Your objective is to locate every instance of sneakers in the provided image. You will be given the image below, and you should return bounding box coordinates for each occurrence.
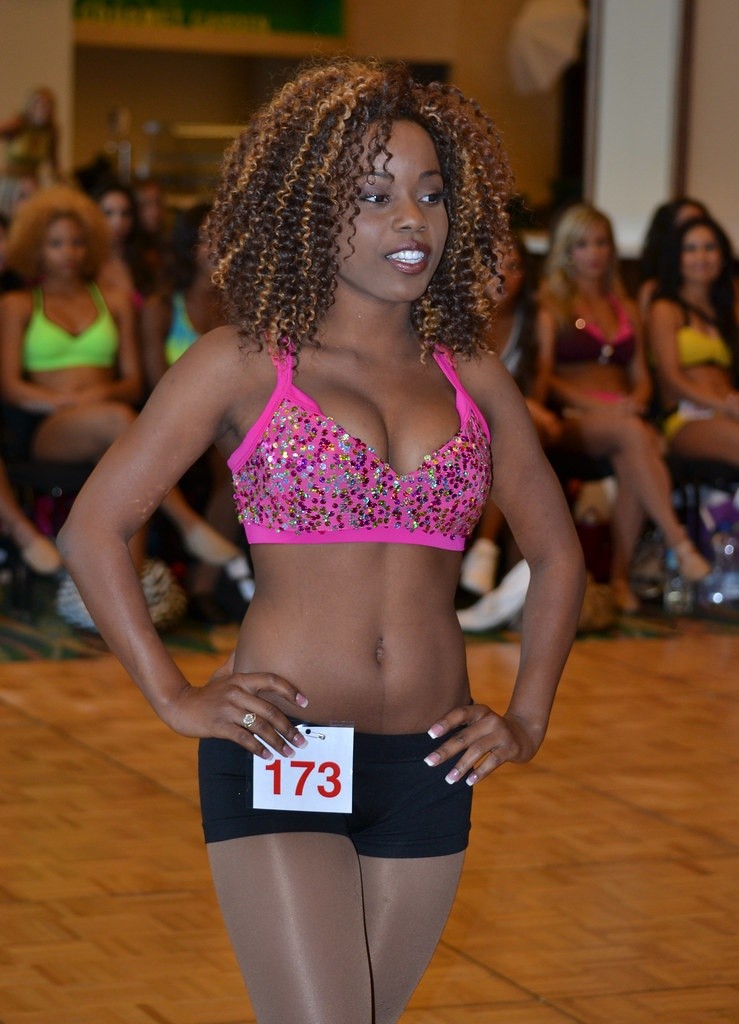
[460,539,500,594]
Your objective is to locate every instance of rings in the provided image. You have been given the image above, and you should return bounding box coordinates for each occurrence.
[243,714,254,726]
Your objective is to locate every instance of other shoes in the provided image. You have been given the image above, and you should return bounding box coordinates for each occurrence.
[183,524,240,566]
[19,533,63,576]
[606,576,640,614]
[666,532,708,581]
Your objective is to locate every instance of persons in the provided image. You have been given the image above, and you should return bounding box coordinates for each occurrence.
[0,86,248,631]
[53,65,587,1023]
[460,197,739,615]
